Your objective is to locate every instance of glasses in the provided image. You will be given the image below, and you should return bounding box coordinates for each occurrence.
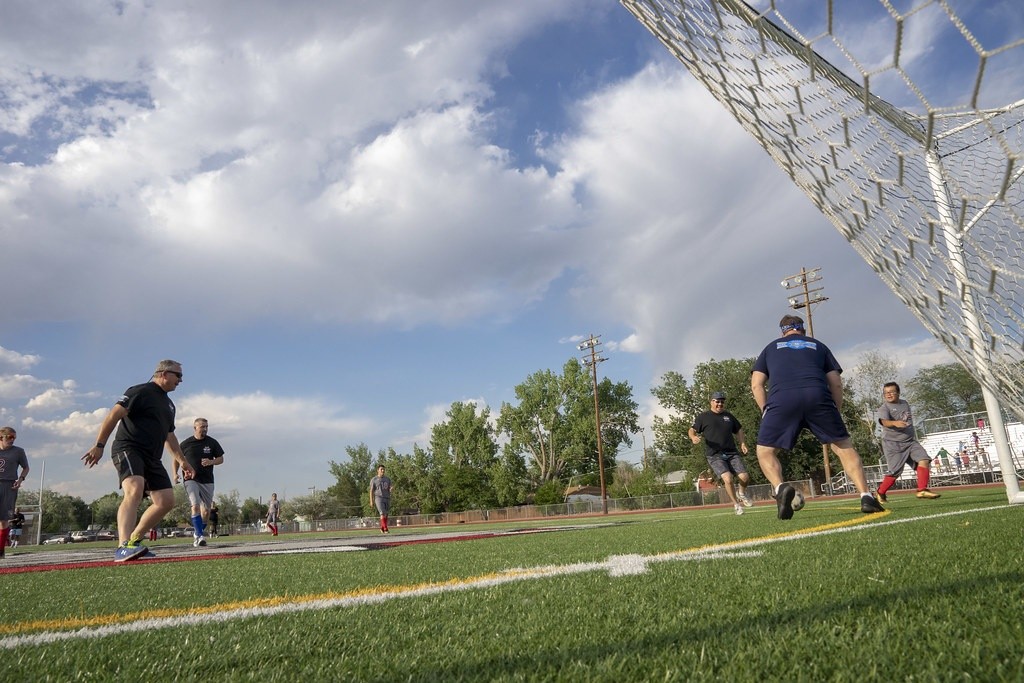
[159,369,183,378]
[884,390,898,396]
[1,434,16,440]
[712,400,724,404]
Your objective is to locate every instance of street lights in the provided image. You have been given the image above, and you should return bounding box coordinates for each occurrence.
[576,333,610,515]
[780,265,833,488]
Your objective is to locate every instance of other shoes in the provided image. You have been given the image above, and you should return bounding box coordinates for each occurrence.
[272,528,275,533]
[380,528,385,533]
[271,533,278,536]
[11,543,14,548]
[384,529,389,533]
[0,550,5,559]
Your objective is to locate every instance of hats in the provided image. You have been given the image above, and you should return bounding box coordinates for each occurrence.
[711,391,726,400]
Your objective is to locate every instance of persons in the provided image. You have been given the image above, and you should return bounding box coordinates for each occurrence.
[688,391,753,515]
[209,501,219,538]
[80,359,196,562]
[173,418,225,547]
[8,507,25,548]
[750,315,884,521]
[265,493,280,536]
[978,418,983,429]
[934,431,984,472]
[369,465,393,534]
[149,527,157,541]
[0,427,29,559]
[875,382,941,503]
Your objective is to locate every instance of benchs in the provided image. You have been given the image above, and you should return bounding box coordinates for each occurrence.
[882,422,1024,480]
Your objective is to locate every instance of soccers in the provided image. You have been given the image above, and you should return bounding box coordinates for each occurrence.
[791,490,804,511]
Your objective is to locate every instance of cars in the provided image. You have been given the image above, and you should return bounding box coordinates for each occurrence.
[42,535,75,544]
[67,530,119,542]
[144,526,195,539]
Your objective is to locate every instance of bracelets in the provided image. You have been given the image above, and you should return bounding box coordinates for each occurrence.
[20,476,25,481]
[96,443,104,448]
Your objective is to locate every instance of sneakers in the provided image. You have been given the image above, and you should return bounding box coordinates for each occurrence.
[875,489,887,504]
[198,535,207,546]
[736,490,753,506]
[734,505,744,515]
[861,493,885,512]
[915,489,941,500]
[128,538,156,559]
[114,540,148,562]
[193,532,199,547]
[770,483,796,520]
